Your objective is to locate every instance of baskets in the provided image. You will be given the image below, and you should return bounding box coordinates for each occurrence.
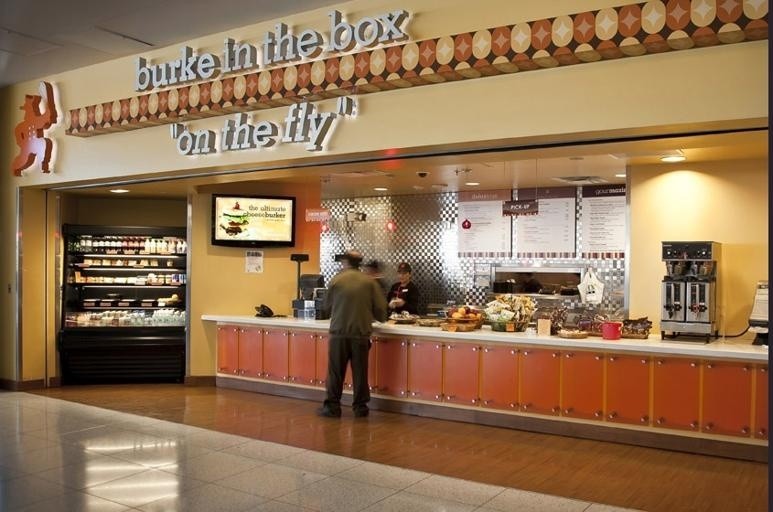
[439,311,487,332]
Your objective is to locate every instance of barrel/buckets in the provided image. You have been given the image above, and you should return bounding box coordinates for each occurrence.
[602,321,624,340]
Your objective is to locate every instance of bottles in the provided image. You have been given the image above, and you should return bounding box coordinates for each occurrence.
[63,235,187,327]
[527,321,538,337]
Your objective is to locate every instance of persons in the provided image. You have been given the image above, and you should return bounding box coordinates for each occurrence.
[313,250,388,419]
[366,260,387,294]
[521,273,543,293]
[387,262,422,315]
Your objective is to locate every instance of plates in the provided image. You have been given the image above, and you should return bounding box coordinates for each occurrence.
[389,313,420,325]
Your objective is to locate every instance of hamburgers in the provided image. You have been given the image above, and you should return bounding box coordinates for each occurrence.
[222,208,248,237]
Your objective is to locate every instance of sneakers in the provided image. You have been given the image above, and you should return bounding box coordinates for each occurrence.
[315,407,339,418]
[352,406,369,417]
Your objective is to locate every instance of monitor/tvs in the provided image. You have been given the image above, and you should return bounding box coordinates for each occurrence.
[300,274,324,300]
[210,193,296,248]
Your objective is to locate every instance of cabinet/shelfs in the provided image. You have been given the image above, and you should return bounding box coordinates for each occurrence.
[55,222,186,385]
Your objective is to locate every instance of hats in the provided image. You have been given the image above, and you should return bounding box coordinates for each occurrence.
[397,263,411,273]
[367,261,378,268]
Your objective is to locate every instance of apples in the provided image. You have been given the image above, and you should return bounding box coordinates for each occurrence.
[448,306,480,319]
